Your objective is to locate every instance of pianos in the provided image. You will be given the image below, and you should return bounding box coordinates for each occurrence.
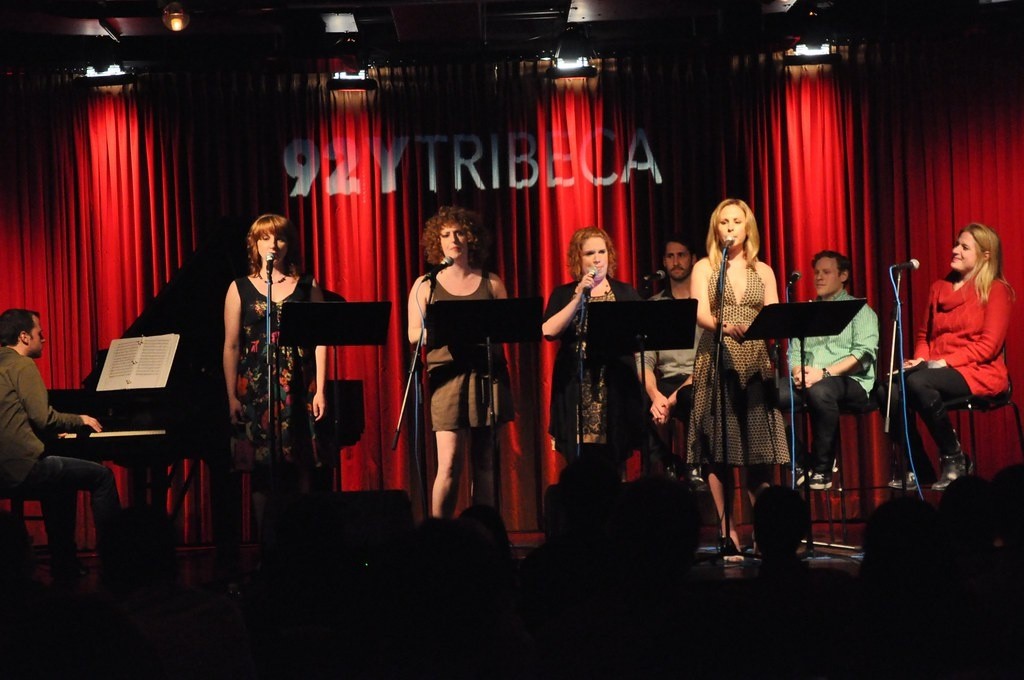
[42,219,365,564]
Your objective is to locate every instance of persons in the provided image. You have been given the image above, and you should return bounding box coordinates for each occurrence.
[635,197,791,562]
[878,222,1014,492]
[0,462,1024,680]
[404,208,515,520]
[1,310,135,595]
[541,227,649,484]
[221,213,329,571]
[775,248,880,490]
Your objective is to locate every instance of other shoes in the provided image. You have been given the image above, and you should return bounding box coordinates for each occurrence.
[888,471,918,490]
[795,464,832,489]
[933,452,973,490]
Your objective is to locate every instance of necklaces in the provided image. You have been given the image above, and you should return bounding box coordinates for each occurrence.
[257,273,287,284]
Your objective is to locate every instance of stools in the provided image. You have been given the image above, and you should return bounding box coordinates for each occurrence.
[649,341,1024,552]
[0,479,57,549]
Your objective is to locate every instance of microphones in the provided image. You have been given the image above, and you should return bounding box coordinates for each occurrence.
[586,267,598,298]
[722,236,735,250]
[643,270,666,282]
[786,270,802,287]
[264,253,275,282]
[894,259,920,270]
[422,256,454,282]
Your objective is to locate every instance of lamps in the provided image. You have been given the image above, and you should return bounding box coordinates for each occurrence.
[327,37,379,91]
[547,29,597,80]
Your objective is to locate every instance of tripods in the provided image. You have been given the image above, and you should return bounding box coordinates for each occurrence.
[692,251,763,566]
[741,297,868,564]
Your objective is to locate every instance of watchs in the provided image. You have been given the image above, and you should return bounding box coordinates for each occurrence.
[822,368,830,379]
